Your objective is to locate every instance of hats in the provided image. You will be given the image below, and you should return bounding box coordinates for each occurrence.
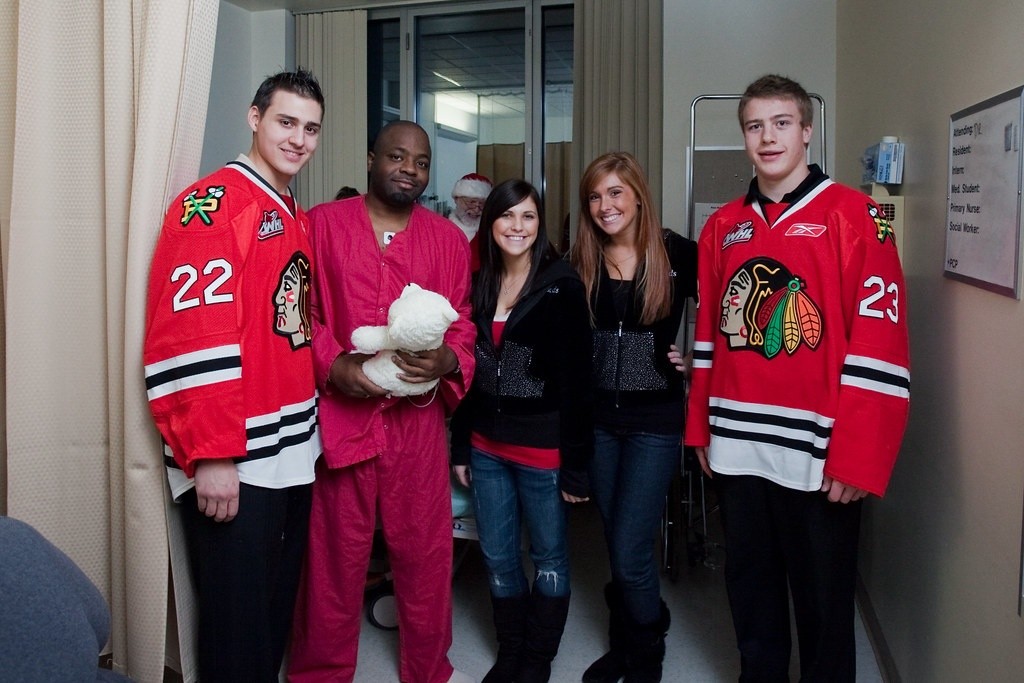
[451,172,494,200]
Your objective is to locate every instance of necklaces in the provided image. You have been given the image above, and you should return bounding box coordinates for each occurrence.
[606,244,642,268]
[500,267,530,296]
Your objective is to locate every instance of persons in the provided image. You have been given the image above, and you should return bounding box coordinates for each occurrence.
[142,63,319,683]
[285,120,478,683]
[446,170,601,683]
[683,72,914,683]
[556,149,705,683]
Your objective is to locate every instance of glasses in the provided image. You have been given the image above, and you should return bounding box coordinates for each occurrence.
[462,196,485,210]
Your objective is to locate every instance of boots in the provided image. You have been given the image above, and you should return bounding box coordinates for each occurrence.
[513,590,573,683]
[582,578,631,683]
[480,579,531,683]
[623,598,670,683]
